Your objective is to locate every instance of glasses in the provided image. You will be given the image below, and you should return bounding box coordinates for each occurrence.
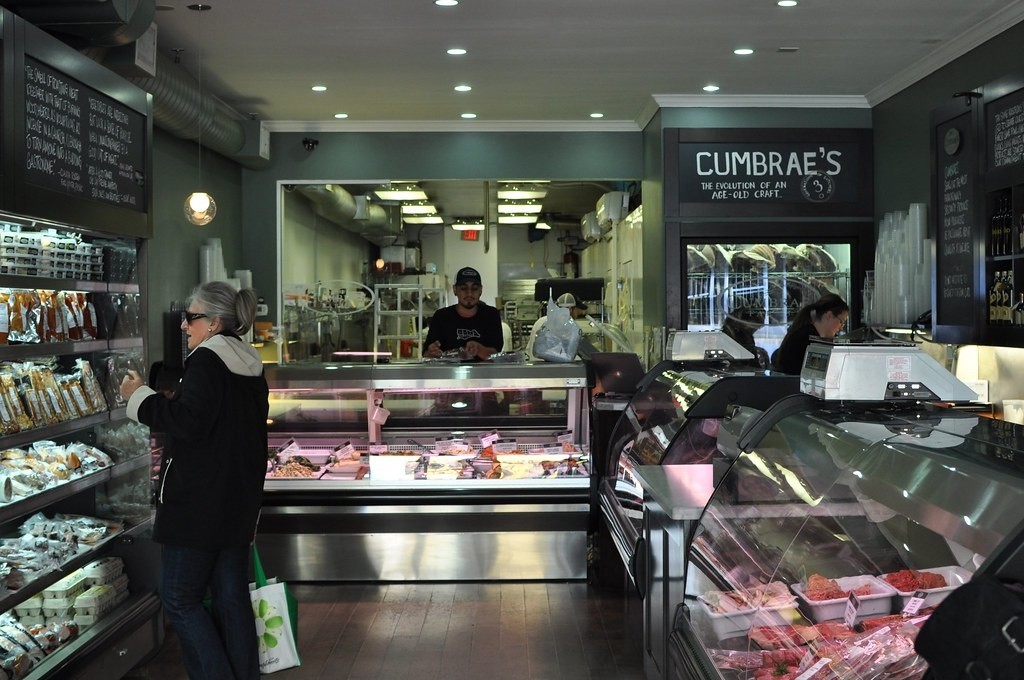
[835,316,846,326]
[185,310,208,324]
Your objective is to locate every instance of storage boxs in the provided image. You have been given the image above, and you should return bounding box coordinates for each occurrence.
[791,574,898,623]
[696,580,800,642]
[877,565,974,611]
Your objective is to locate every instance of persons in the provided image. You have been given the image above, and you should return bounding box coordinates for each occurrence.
[721,302,767,366]
[119,281,270,680]
[417,293,589,364]
[780,294,850,375]
[419,266,504,359]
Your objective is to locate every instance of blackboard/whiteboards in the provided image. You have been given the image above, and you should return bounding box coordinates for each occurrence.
[9,14,154,239]
[929,85,986,345]
[984,87,1024,192]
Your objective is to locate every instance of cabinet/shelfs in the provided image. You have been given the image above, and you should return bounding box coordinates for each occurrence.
[928,67,1024,349]
[581,204,644,367]
[373,283,444,362]
[0,273,166,680]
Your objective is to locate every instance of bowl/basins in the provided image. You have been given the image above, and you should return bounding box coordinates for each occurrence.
[199,238,252,293]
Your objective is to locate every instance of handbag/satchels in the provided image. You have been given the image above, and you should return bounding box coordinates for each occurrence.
[203,542,300,675]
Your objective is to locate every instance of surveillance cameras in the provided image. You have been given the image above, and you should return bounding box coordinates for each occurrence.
[284,184,296,191]
[303,139,319,151]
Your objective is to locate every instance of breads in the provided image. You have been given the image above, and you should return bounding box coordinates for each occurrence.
[1,449,81,480]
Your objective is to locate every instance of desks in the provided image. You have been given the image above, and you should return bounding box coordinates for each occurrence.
[510,319,538,351]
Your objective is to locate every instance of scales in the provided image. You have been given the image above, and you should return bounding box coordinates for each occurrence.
[800,335,978,403]
[666,330,754,360]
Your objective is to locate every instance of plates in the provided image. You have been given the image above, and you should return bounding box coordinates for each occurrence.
[264,465,369,480]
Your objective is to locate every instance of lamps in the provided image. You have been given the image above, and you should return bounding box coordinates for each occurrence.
[374,183,444,224]
[497,180,552,230]
[451,218,486,231]
[183,3,217,225]
[376,259,385,268]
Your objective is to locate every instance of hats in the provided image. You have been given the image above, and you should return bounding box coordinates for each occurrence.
[557,293,588,310]
[454,268,481,286]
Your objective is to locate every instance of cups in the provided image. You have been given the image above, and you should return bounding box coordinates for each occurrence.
[1003,400,1024,426]
[401,341,413,357]
